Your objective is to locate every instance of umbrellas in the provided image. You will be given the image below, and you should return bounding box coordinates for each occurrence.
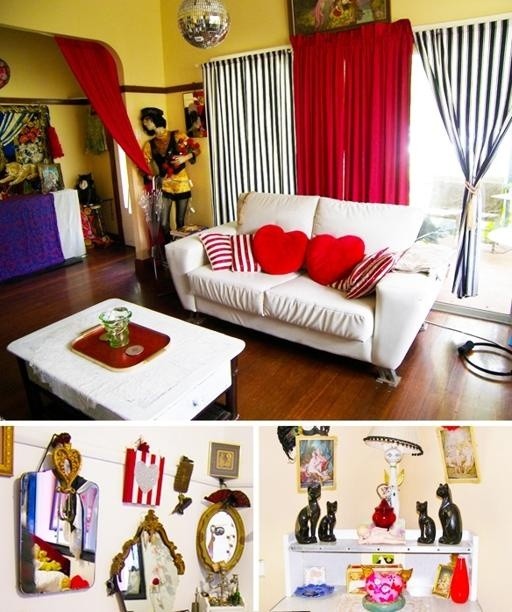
[138,174,163,280]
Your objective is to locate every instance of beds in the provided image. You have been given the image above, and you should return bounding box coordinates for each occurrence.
[6,297,246,421]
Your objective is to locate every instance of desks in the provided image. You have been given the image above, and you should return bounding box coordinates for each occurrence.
[0,188,87,286]
[270,528,484,612]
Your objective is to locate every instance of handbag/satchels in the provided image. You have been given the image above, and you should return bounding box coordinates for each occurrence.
[123,439,165,505]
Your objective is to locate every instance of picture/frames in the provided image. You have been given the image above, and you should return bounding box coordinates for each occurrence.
[287,0,391,36]
[436,426,481,484]
[208,441,241,480]
[295,436,337,493]
[37,163,64,195]
[0,426,14,478]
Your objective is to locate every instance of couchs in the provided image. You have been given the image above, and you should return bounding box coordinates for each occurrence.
[164,192,451,386]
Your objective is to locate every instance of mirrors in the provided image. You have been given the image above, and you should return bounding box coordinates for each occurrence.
[183,91,208,138]
[196,502,245,574]
[111,509,185,612]
[20,472,99,596]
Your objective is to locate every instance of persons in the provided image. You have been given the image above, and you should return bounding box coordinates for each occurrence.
[308,451,325,477]
[140,108,201,242]
[77,173,97,207]
[187,112,207,138]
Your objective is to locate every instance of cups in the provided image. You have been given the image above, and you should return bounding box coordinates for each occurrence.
[99,307,134,349]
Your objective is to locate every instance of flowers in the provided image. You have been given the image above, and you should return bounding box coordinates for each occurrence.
[16,117,49,163]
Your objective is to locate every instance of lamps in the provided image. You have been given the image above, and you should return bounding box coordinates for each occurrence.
[358,425,423,547]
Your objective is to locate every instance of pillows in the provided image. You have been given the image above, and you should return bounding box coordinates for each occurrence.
[196,224,425,299]
